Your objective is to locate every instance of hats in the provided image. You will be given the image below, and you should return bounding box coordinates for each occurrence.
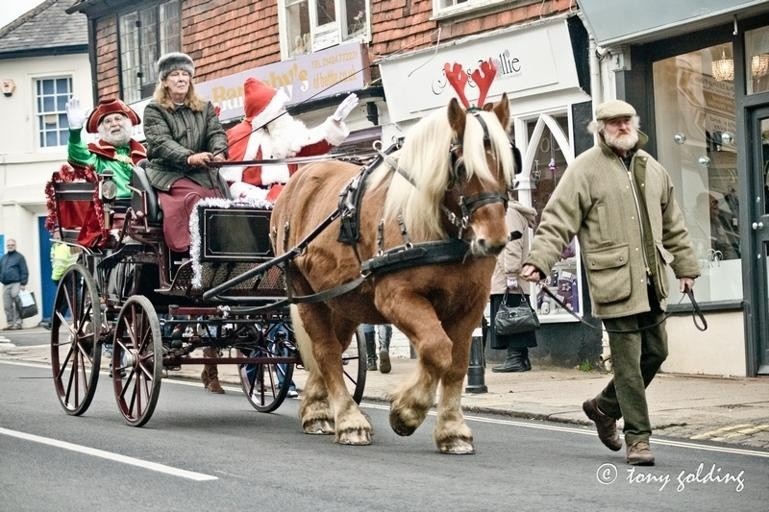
[86,97,140,133]
[158,52,195,81]
[595,99,636,121]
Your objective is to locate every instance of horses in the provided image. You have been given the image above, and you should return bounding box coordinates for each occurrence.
[270,92,517,454]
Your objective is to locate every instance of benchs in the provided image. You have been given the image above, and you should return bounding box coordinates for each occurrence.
[127,158,174,289]
[50,171,104,257]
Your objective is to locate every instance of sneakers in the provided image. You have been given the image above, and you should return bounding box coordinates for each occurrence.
[627,441,654,465]
[277,388,298,397]
[3,326,22,331]
[583,398,622,451]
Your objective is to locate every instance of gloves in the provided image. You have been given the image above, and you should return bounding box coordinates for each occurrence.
[506,276,518,289]
[65,97,90,130]
[332,93,359,123]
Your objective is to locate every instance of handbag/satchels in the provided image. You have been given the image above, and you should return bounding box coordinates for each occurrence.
[494,302,540,337]
[14,291,38,319]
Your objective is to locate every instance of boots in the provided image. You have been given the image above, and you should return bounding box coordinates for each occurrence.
[491,348,531,373]
[201,347,225,394]
[364,331,377,371]
[378,325,392,373]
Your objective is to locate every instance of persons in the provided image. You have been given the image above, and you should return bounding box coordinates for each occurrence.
[519,99,701,465]
[488,202,538,373]
[363,322,393,374]
[0,238,28,331]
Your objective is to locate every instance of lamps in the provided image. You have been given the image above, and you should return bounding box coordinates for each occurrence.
[711,44,734,82]
[749,52,768,82]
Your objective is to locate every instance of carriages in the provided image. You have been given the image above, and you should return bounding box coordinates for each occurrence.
[47,91,517,457]
